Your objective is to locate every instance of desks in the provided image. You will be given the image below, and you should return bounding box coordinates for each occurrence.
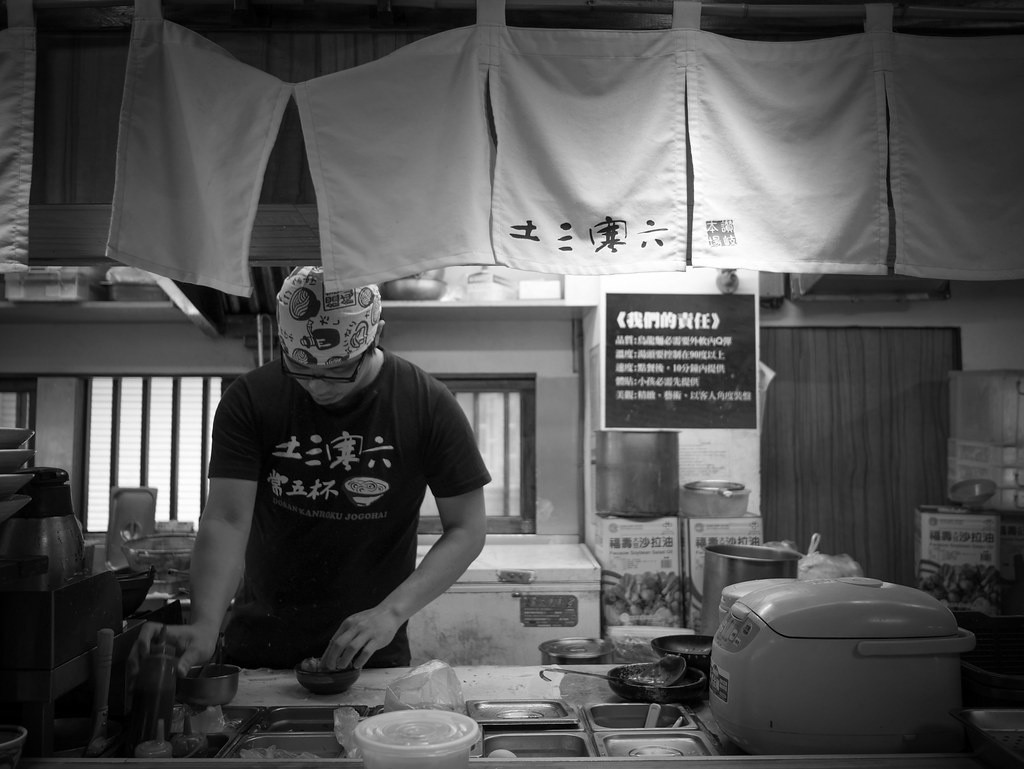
[230,662,747,755]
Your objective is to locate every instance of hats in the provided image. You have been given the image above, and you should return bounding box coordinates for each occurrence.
[276,266,381,368]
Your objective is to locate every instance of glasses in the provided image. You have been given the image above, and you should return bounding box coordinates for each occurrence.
[279,344,366,384]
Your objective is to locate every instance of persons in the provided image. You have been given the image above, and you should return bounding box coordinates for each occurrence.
[126,264,490,679]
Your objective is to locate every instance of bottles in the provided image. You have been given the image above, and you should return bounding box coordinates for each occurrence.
[127,643,178,758]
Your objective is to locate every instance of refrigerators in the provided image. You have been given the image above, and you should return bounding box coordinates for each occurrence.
[406,542,600,668]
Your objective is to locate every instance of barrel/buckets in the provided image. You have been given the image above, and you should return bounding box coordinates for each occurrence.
[701,543,803,637]
[537,635,615,667]
[594,427,681,518]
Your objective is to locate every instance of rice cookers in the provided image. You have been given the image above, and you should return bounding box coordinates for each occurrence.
[709,577,977,756]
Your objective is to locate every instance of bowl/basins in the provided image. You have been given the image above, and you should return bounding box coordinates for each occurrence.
[295,658,362,696]
[0,448,36,474]
[651,634,714,674]
[121,533,196,583]
[0,474,35,501]
[679,479,751,518]
[0,725,28,769]
[0,494,36,524]
[180,663,241,706]
[384,269,447,300]
[0,427,36,449]
[607,664,706,707]
[352,708,481,768]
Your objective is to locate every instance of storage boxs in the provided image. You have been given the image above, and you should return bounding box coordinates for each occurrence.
[914,508,1001,615]
[684,513,764,627]
[949,369,1024,511]
[593,515,679,628]
